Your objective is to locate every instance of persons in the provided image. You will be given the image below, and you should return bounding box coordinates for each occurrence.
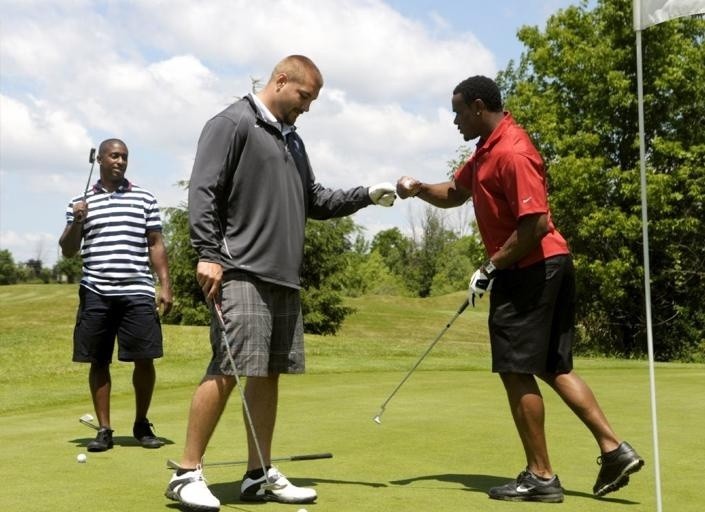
[164,54,399,511]
[58,137,175,452]
[395,74,645,503]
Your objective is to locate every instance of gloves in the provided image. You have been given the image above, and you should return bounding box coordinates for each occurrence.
[486,470,565,502]
[468,258,496,309]
[367,182,398,207]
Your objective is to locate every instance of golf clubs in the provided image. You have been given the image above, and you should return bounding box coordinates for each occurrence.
[374,294,473,425]
[77,149,96,220]
[168,450,332,471]
[79,414,100,431]
[211,294,287,491]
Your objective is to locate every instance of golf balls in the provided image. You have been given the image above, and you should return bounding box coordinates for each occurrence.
[77,454,86,463]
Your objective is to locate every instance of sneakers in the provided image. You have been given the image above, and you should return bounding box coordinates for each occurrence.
[164,465,223,511]
[130,410,163,450]
[240,468,319,504]
[86,424,113,453]
[593,439,646,497]
